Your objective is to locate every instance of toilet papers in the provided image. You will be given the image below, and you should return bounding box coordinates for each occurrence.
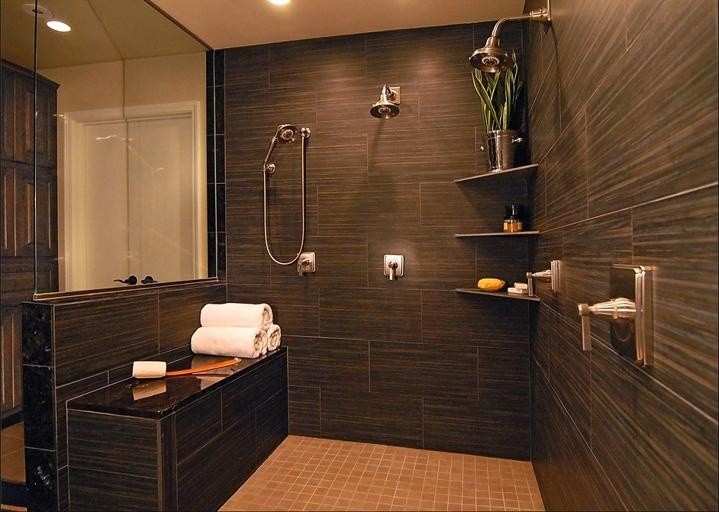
[131,379,167,401]
[131,360,167,378]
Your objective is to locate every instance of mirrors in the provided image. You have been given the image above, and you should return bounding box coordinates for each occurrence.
[34,2,218,299]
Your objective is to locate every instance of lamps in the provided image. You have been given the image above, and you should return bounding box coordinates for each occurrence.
[371,83,401,119]
[469,0,552,74]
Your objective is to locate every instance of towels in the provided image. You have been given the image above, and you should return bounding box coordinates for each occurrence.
[191,301,281,361]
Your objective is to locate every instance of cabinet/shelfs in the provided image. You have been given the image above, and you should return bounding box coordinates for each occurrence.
[454,164,540,301]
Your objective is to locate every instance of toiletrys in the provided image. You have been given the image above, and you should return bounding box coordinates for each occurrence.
[503,199,527,233]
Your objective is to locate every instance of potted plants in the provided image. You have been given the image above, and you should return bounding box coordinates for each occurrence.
[472,53,523,172]
